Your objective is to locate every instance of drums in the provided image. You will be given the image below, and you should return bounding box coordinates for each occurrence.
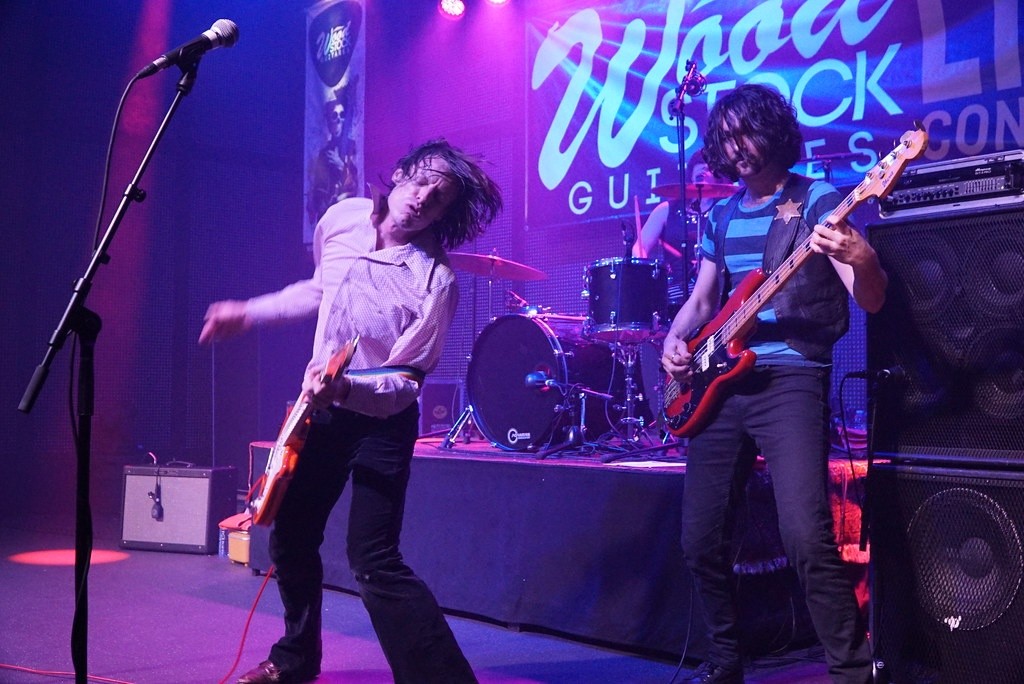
[582,256,671,346]
[465,313,625,454]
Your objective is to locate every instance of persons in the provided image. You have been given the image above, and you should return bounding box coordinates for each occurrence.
[632,147,733,455]
[659,84,889,684]
[198,137,504,684]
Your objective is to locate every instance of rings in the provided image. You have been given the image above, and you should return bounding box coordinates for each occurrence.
[672,353,680,363]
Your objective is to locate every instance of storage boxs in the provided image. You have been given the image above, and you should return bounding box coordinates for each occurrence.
[117,462,238,555]
[228,531,250,567]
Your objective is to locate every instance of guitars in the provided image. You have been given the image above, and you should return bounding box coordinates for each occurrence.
[657,119,928,439]
[248,336,360,527]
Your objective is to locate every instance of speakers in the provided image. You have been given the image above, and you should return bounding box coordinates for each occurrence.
[869,464,1024,684]
[862,201,1024,470]
[119,464,239,555]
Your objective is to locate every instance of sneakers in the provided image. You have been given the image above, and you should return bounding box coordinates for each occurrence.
[681,663,744,684]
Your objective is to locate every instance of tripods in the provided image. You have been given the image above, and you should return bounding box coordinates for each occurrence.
[535,341,688,460]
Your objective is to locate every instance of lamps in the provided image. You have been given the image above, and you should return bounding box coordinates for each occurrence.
[438,0,472,21]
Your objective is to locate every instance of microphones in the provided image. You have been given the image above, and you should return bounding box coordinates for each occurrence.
[137,19,240,80]
[620,222,632,245]
[525,372,556,388]
[150,486,163,518]
[688,75,707,94]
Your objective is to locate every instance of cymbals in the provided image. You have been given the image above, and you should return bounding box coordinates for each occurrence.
[445,251,549,281]
[651,183,743,198]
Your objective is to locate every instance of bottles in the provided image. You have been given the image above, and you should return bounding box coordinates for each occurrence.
[219,527,229,557]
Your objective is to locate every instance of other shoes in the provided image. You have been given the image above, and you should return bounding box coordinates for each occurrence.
[239,658,323,684]
